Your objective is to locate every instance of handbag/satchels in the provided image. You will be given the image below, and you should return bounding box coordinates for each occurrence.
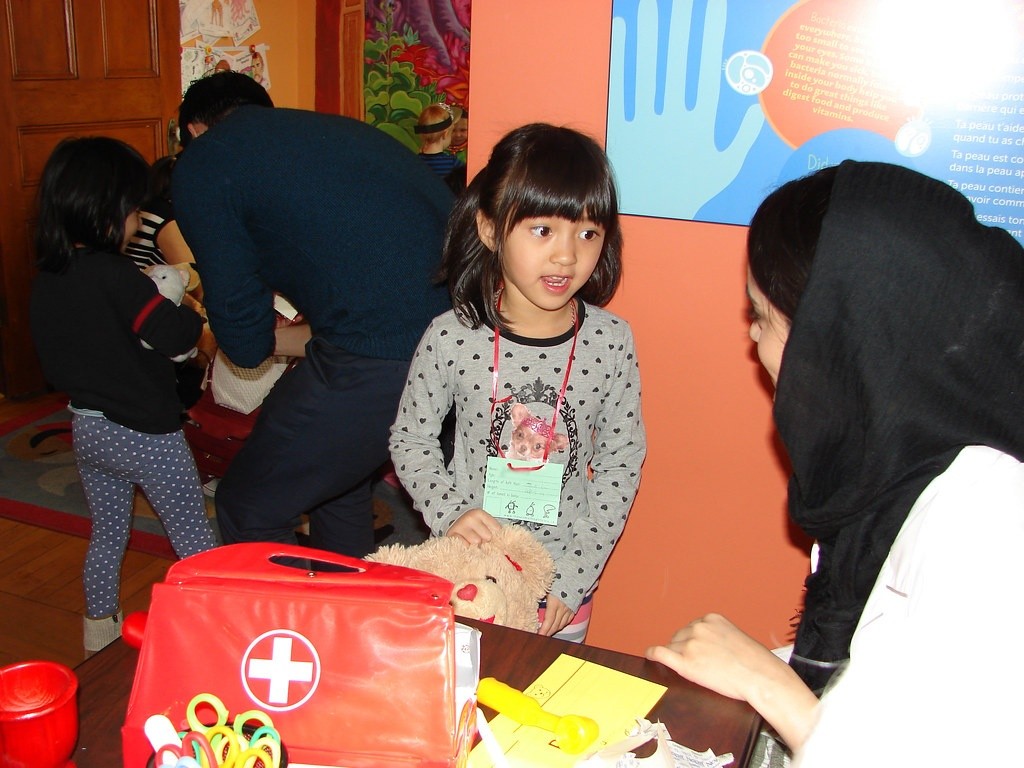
[120,541,479,768]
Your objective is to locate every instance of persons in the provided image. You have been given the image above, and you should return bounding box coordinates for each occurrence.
[35,137,218,650]
[388,123,647,644]
[647,160,1024,768]
[125,157,197,273]
[172,73,467,573]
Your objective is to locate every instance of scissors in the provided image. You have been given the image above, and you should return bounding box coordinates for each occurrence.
[153,692,281,768]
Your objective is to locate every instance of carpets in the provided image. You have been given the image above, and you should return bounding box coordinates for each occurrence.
[0,397,455,571]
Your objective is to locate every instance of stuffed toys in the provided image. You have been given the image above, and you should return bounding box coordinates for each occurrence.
[141,262,199,360]
[357,527,555,634]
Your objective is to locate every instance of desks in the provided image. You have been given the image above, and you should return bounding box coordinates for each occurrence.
[70,615,763,768]
